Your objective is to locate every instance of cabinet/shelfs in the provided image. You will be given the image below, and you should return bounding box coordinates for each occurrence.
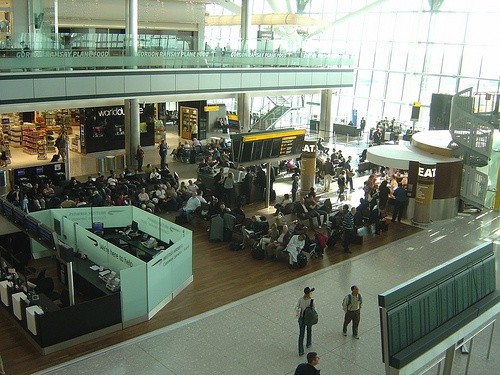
[180,106,198,140]
[46,128,61,153]
[22,130,37,155]
[37,129,47,160]
[45,114,55,126]
[0,125,11,164]
[10,129,22,148]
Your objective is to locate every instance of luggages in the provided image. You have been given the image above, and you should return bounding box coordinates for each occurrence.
[51,152,60,162]
[326,226,344,247]
[209,216,224,242]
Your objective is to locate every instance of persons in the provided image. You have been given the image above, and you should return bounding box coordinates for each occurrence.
[0,43,414,269]
[295,287,315,356]
[342,285,362,340]
[293,352,320,375]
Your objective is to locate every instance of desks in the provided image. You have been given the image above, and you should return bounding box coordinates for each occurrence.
[121,236,162,255]
[103,232,122,240]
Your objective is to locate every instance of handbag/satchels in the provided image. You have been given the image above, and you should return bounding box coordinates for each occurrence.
[252,247,264,259]
[175,215,187,224]
[223,227,232,241]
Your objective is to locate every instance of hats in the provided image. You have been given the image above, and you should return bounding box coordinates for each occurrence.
[304,287,315,294]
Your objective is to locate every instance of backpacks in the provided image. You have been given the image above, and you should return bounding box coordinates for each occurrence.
[303,299,318,325]
[342,294,360,307]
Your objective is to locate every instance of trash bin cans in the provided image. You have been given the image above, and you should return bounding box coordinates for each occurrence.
[96,156,106,175]
[115,153,125,170]
[105,155,116,172]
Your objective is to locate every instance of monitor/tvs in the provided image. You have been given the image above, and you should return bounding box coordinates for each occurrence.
[131,221,138,233]
[0,198,60,258]
[26,281,37,295]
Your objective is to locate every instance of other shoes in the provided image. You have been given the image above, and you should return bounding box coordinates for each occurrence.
[343,333,347,336]
[299,352,305,356]
[354,335,359,339]
[138,168,143,172]
[241,246,245,249]
[240,244,243,245]
[306,345,312,348]
[319,226,322,228]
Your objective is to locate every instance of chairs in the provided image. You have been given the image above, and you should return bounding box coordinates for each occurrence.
[72,144,383,268]
[333,123,362,137]
